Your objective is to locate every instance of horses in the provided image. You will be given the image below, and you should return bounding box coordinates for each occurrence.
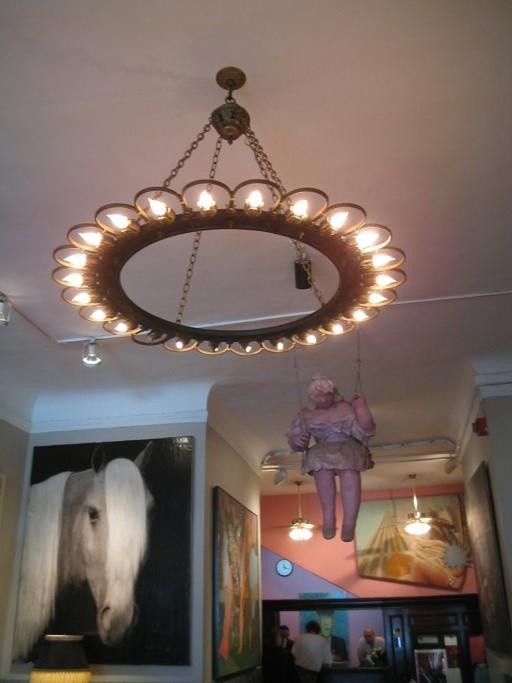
[12,440,159,662]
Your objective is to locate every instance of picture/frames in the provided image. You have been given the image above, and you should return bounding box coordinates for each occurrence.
[212,483,261,681]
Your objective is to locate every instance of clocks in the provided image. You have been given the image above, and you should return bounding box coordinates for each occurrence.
[275,557,294,578]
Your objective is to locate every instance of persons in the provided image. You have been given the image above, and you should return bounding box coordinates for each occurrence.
[288,374,377,543]
[320,617,348,662]
[356,627,386,667]
[263,628,297,683]
[291,620,332,683]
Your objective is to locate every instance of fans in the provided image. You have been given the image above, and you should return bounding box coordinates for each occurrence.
[382,472,459,531]
[269,480,340,531]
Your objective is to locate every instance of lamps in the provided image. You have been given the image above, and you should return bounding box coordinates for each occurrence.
[49,64,411,358]
[79,336,105,370]
